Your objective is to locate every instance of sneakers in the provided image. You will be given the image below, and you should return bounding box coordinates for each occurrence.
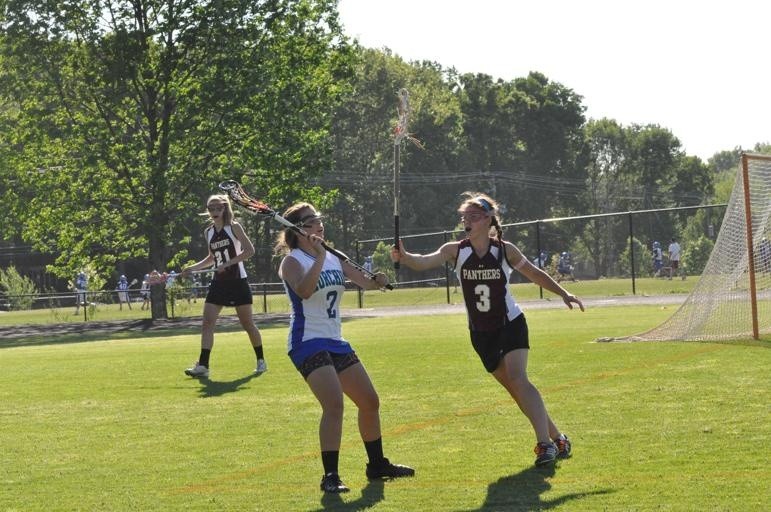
[365,456,416,481]
[183,363,210,380]
[320,471,351,493]
[530,439,560,468]
[253,359,268,375]
[553,434,573,460]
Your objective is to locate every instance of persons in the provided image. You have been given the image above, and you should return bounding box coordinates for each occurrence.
[185,204,268,377]
[557,252,571,274]
[533,252,546,268]
[390,191,585,466]
[75,270,179,309]
[272,203,414,492]
[362,256,373,276]
[668,238,681,271]
[651,241,663,272]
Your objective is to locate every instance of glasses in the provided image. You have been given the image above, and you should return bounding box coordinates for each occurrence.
[208,203,225,214]
[460,211,487,223]
